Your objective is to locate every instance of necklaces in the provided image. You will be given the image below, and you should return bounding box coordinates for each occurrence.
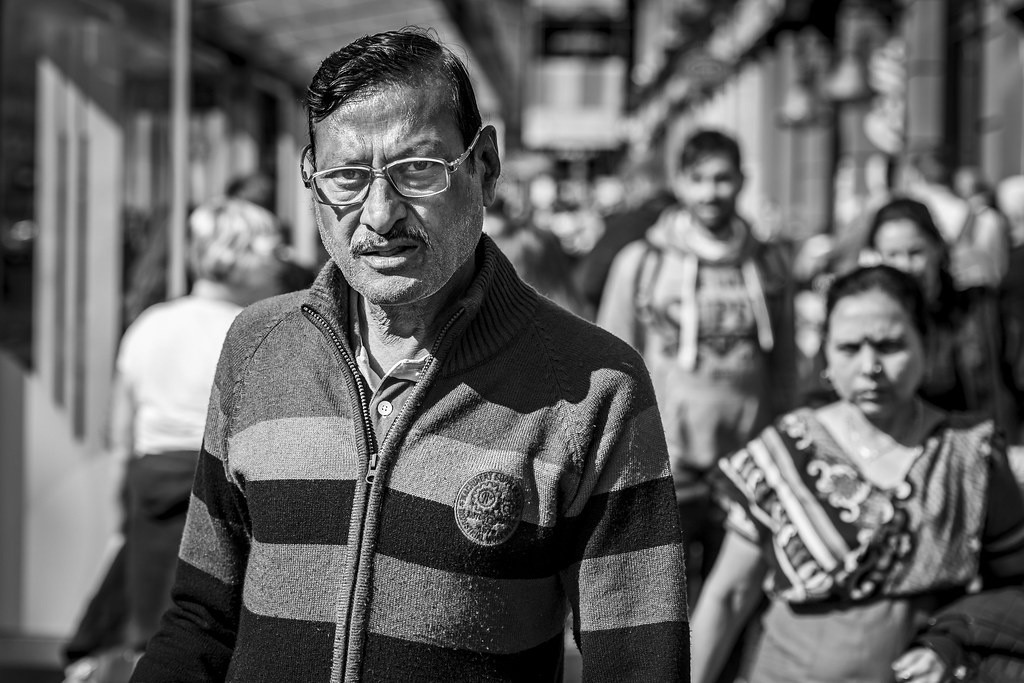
[838,391,923,459]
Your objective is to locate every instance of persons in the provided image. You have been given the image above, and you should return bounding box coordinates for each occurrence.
[474,153,1024,592]
[594,123,788,602]
[128,30,692,683]
[690,267,1024,683]
[0,175,322,376]
[115,194,313,668]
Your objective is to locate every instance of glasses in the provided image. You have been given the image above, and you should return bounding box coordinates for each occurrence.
[298,136,482,203]
